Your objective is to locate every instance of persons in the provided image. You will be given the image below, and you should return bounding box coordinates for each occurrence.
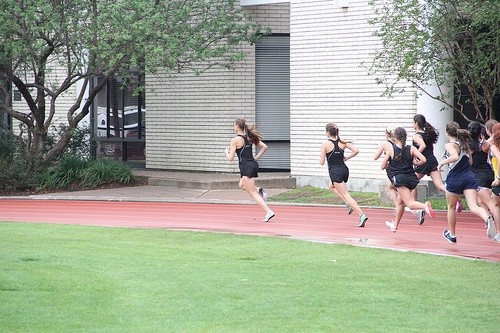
[319,122,368,227]
[437,120,497,244]
[224,118,276,222]
[380,126,436,233]
[374,124,425,225]
[469,119,500,242]
[405,114,463,214]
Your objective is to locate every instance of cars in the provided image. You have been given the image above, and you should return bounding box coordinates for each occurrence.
[97,106,147,156]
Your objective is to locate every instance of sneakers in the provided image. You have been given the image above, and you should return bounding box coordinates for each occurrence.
[418,209,425,225]
[456,196,462,212]
[386,220,398,233]
[443,229,456,244]
[358,214,368,227]
[257,187,263,198]
[486,216,496,239]
[262,211,275,221]
[346,203,354,215]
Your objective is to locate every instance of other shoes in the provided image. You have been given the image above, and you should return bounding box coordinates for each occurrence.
[425,201,436,219]
[493,234,500,241]
[404,207,418,215]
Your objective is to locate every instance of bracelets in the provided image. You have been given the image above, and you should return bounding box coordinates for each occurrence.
[225,151,230,156]
